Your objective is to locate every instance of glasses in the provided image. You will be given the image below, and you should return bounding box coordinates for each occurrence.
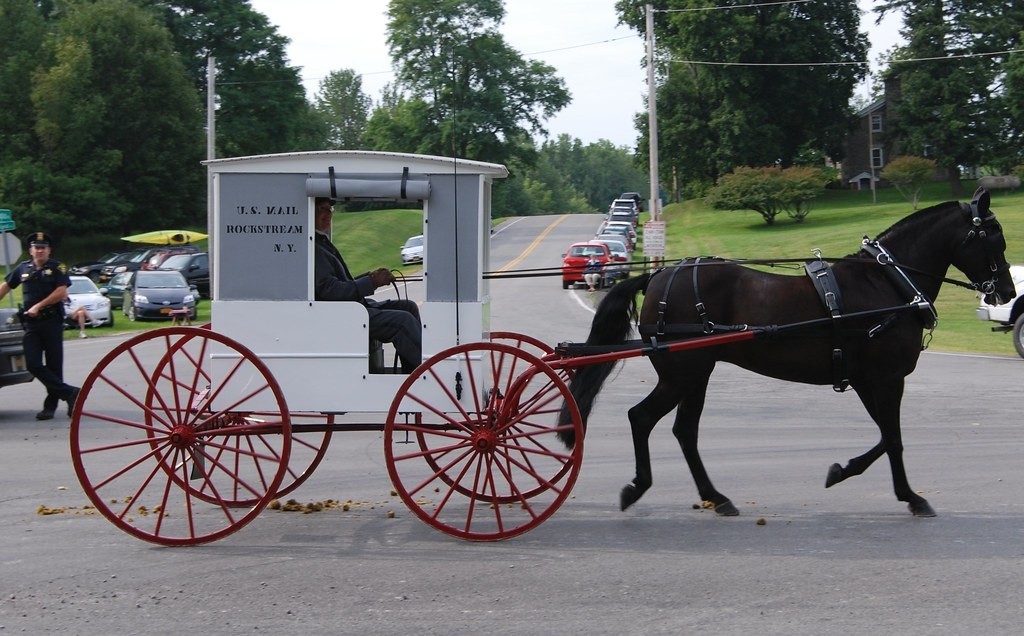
[316,205,334,212]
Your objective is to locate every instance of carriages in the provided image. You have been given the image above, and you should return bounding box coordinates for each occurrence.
[67,148,1020,550]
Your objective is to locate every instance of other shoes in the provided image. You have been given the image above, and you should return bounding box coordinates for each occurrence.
[588,287,595,292]
[79,332,87,338]
[92,320,104,328]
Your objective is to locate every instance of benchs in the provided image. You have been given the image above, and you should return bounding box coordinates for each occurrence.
[582,257,629,290]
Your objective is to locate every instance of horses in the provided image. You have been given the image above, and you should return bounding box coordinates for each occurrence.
[554,186,1017,518]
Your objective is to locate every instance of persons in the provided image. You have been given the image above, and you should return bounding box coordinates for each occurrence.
[64,298,104,338]
[0,233,81,419]
[582,255,602,292]
[602,255,621,287]
[314,198,422,374]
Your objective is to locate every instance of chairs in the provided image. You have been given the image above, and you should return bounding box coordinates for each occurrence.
[575,247,583,256]
[63,317,92,338]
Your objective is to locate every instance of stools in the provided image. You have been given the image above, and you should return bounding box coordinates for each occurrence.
[168,309,192,326]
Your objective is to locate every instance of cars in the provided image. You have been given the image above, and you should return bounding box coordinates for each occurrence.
[122,270,197,322]
[581,192,645,279]
[491,215,494,233]
[69,244,210,309]
[400,234,425,266]
[975,265,1024,359]
[60,275,114,328]
[561,241,612,289]
[0,307,35,388]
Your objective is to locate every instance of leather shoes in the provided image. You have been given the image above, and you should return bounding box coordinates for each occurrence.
[68,387,80,417]
[36,409,54,419]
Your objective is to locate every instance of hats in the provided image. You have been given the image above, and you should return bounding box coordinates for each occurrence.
[315,197,336,206]
[26,232,52,249]
